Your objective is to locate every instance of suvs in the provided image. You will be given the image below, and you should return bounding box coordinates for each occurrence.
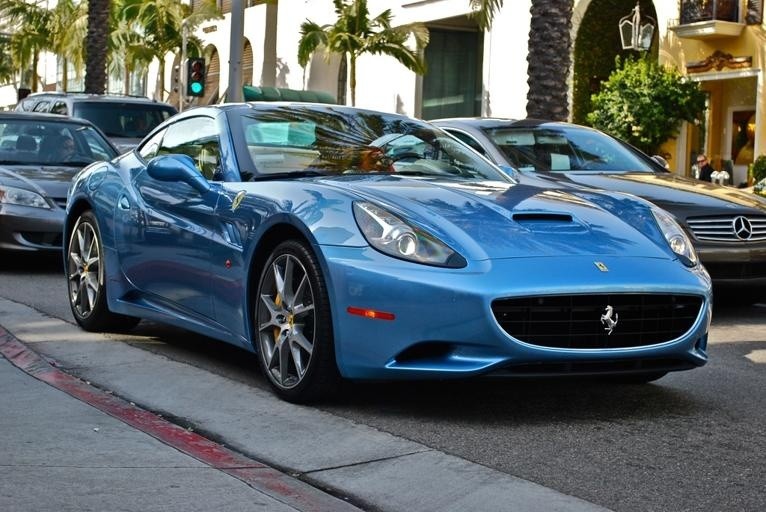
[12,91,181,158]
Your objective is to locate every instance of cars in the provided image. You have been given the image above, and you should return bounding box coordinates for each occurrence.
[361,117,766,288]
[0,111,123,254]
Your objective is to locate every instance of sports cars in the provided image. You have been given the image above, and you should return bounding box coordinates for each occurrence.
[61,100,715,409]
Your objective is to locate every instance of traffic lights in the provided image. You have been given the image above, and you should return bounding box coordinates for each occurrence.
[183,57,206,97]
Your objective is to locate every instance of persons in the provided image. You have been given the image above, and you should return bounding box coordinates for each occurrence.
[53,133,77,162]
[695,154,716,182]
[308,121,358,174]
